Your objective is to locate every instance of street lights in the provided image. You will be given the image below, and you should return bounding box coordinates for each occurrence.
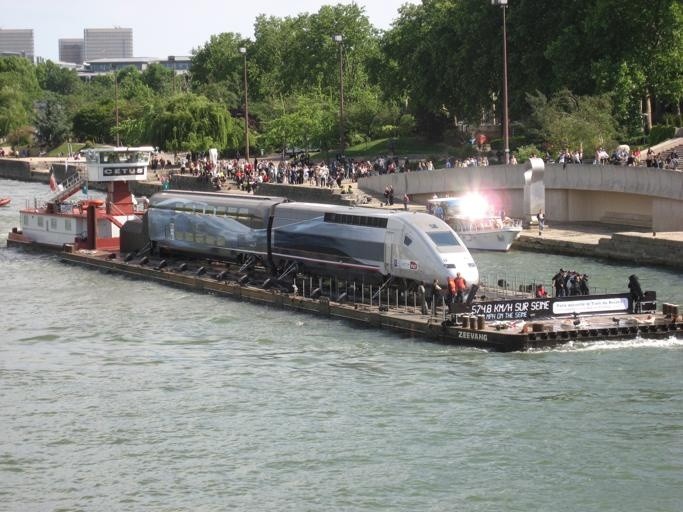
[115,68,131,147]
[238,46,249,162]
[332,34,345,157]
[491,0,510,165]
[168,55,175,91]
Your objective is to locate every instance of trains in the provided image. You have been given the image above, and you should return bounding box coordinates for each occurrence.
[82,56,192,76]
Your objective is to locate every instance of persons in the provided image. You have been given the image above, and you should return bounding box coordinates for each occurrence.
[628,274,643,314]
[149,144,345,197]
[535,284,547,298]
[383,186,389,206]
[536,209,544,236]
[446,275,457,314]
[496,150,504,161]
[593,143,681,170]
[453,271,467,303]
[430,279,441,315]
[344,153,489,179]
[388,185,393,205]
[433,204,443,220]
[550,266,590,299]
[0,144,145,165]
[418,280,428,316]
[529,148,584,171]
[401,193,409,210]
[509,154,517,165]
